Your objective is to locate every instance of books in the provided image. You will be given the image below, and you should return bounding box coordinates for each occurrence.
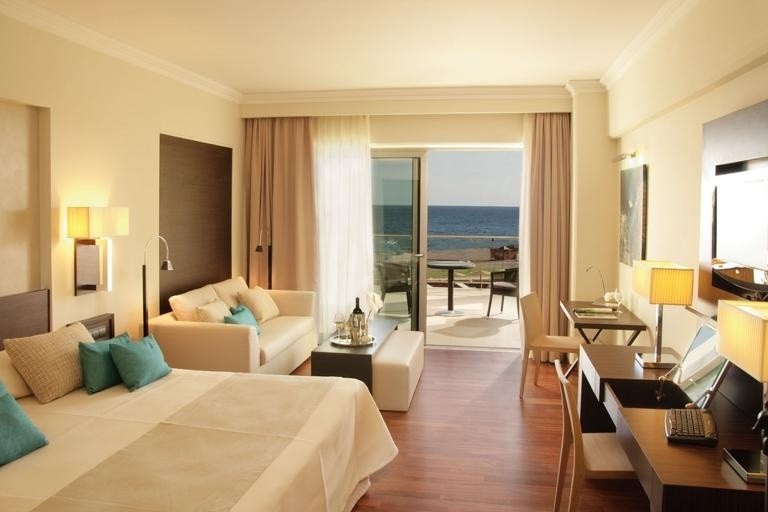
[573,307,617,320]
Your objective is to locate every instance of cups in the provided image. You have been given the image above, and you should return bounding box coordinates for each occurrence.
[357,312,369,339]
[603,292,618,309]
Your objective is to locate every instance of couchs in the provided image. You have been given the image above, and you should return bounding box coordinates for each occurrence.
[147,276,318,375]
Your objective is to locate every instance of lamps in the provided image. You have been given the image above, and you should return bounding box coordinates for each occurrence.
[631,259,693,371]
[253,226,271,289]
[714,298,767,485]
[66,205,130,295]
[143,234,174,336]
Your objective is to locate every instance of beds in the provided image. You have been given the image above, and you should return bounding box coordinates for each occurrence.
[0,288,399,512]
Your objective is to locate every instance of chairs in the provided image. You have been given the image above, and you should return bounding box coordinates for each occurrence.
[554,357,640,512]
[376,262,413,315]
[519,290,580,400]
[487,268,519,317]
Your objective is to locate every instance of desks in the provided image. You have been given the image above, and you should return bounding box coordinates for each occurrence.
[575,343,768,511]
[559,299,646,378]
[427,260,476,317]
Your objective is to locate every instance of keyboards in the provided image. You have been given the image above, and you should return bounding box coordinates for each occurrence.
[665,407,720,448]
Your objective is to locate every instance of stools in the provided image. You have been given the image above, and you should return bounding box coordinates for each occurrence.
[369,329,424,412]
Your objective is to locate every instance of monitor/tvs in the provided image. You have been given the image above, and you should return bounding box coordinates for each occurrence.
[711,158,768,301]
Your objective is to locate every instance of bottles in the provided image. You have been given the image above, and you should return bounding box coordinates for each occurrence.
[351,296,363,342]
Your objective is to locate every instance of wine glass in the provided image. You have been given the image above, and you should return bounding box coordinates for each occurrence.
[333,312,349,342]
[613,287,624,315]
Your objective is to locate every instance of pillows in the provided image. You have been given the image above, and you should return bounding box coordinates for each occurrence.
[0,380,48,468]
[3,322,95,405]
[79,332,130,395]
[169,276,280,336]
[109,334,172,393]
